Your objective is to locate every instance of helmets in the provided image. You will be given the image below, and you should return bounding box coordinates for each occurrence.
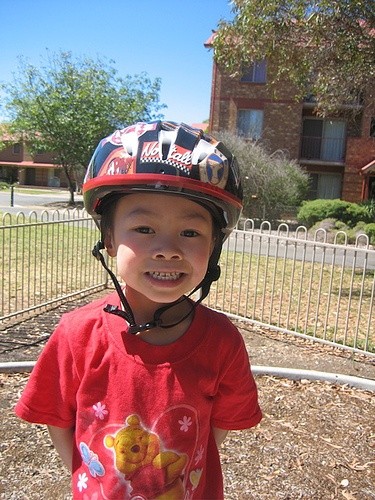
[84,120,244,244]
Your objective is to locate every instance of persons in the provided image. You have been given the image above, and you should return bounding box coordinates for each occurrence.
[13,118,265,500]
